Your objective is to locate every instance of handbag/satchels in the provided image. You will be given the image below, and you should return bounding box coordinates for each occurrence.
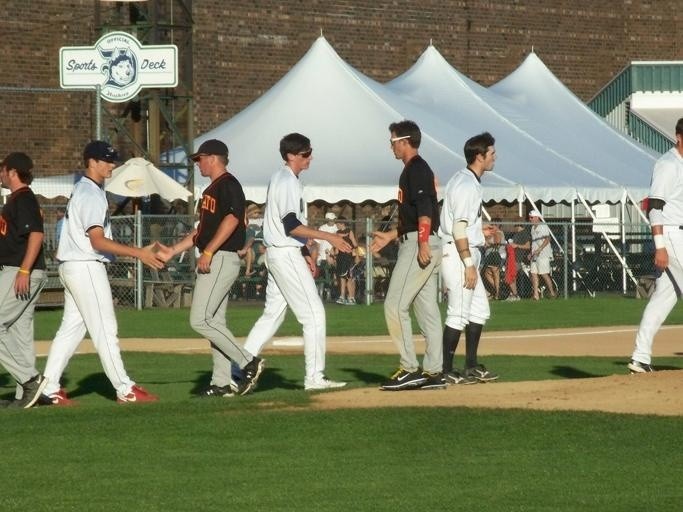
[350,262,364,277]
[485,251,502,266]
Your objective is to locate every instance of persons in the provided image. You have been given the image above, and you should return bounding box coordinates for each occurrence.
[627,117,682,372]
[238,202,265,296]
[368,119,447,389]
[437,132,500,383]
[0,151,50,409]
[307,214,400,304]
[481,210,557,301]
[41,140,167,406]
[35,193,71,272]
[111,193,195,304]
[230,132,352,391]
[144,140,267,398]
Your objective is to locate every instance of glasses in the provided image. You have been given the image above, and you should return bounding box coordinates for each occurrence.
[296,147,312,158]
[390,135,411,145]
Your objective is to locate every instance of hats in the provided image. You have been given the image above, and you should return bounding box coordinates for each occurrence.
[189,140,228,162]
[0,153,32,169]
[84,142,124,164]
[248,204,262,215]
[529,210,538,217]
[325,213,338,219]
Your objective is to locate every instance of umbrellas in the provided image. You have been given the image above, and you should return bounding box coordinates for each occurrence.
[0,158,194,203]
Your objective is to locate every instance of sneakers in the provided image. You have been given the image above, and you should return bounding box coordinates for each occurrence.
[304,375,348,390]
[336,297,357,305]
[381,368,423,390]
[240,357,266,396]
[489,292,557,301]
[420,372,447,388]
[38,389,82,406]
[116,385,160,404]
[192,383,234,398]
[464,364,497,381]
[442,368,477,385]
[628,358,653,373]
[18,375,49,408]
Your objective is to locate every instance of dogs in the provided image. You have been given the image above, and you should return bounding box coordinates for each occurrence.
[109,46,136,86]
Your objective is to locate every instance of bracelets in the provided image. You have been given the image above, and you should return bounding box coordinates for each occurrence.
[203,250,212,258]
[653,235,667,248]
[19,270,29,273]
[417,224,430,242]
[463,258,474,265]
